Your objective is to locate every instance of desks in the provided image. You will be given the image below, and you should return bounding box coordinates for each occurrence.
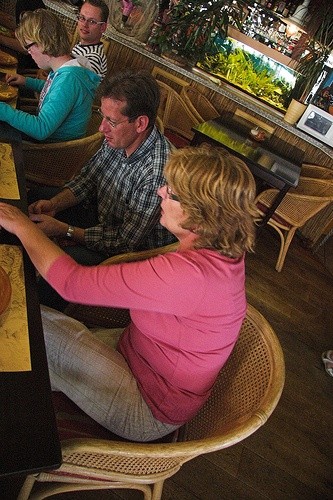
[188,114,305,236]
[0,48,62,500]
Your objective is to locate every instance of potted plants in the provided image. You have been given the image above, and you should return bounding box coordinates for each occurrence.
[144,0,250,67]
[280,0,333,125]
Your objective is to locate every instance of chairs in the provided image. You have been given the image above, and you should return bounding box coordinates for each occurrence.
[15,63,333,499]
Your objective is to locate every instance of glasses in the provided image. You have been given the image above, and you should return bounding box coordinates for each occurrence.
[77,15,105,26]
[22,42,36,51]
[163,183,180,203]
[97,109,138,127]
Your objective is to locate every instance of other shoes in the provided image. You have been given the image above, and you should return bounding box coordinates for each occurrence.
[38,275,61,306]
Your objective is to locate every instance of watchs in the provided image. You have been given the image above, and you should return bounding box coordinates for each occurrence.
[62,225,74,240]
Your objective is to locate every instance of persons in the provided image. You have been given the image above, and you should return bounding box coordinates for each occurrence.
[20,0,108,99]
[0,0,47,69]
[27,68,178,268]
[0,142,258,441]
[0,8,100,144]
